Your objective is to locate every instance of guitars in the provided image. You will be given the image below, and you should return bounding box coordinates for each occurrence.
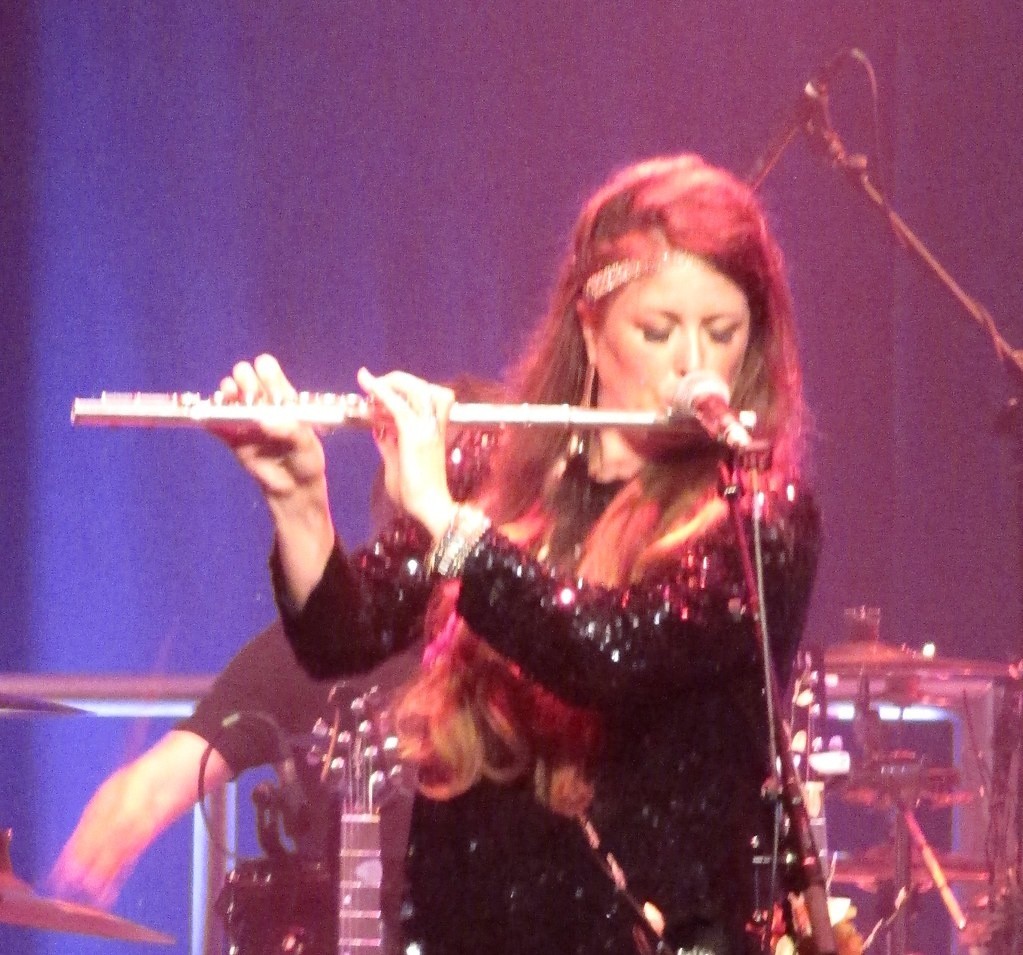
[303,676,408,955]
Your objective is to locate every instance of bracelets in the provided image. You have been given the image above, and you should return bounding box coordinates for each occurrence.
[429,503,492,578]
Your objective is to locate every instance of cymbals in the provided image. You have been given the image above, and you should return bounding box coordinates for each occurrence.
[1,873,177,947]
[818,639,983,680]
[829,844,988,881]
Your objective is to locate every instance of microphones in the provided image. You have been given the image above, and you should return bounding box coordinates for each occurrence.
[673,369,752,449]
[745,48,856,193]
[270,731,312,813]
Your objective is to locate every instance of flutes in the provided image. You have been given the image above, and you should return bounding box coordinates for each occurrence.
[66,387,763,430]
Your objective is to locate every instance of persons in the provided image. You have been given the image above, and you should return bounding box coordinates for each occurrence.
[44,603,343,909]
[211,154,823,955]
